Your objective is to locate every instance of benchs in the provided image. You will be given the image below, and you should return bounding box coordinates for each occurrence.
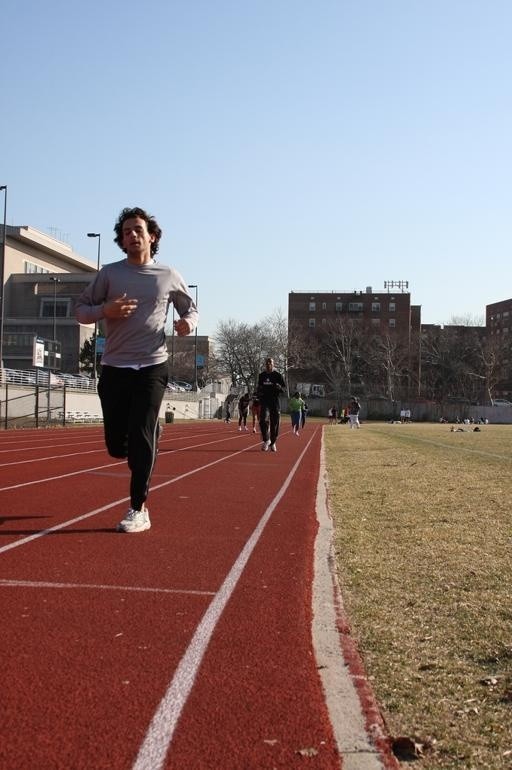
[58,411,103,423]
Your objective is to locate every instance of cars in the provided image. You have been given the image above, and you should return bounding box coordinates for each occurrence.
[0,368,99,389]
[166,381,192,392]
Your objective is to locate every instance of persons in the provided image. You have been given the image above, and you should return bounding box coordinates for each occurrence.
[237,392,250,431]
[439,414,489,425]
[299,393,309,429]
[405,407,411,424]
[251,391,261,433]
[72,206,200,534]
[288,391,305,436]
[400,407,405,424]
[256,357,287,452]
[225,400,232,423]
[328,396,362,430]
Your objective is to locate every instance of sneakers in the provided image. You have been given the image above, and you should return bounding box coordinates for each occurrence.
[117,508,151,533]
[237,426,256,433]
[261,440,277,453]
[292,427,299,436]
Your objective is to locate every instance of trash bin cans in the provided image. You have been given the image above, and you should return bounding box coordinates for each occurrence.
[165,411,174,423]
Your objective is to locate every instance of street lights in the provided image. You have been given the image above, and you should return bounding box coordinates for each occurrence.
[48,277,61,340]
[0,185,6,388]
[88,233,100,379]
[188,285,197,390]
